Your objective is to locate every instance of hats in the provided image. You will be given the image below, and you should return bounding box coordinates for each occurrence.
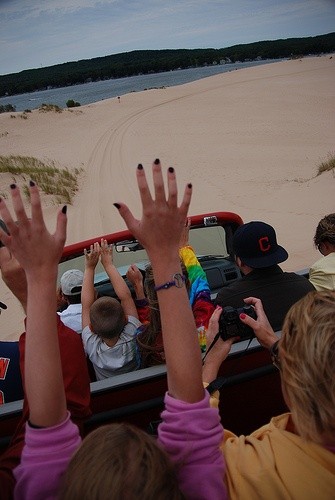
[228,222,289,270]
[58,268,87,296]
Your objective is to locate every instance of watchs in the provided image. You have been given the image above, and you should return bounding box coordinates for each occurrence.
[154,273,188,293]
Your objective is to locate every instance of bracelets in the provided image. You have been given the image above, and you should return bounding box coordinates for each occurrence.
[269,338,281,358]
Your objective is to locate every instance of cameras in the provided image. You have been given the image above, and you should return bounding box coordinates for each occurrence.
[218,305,257,344]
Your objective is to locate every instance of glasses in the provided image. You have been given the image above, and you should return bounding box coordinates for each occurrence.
[266,338,286,370]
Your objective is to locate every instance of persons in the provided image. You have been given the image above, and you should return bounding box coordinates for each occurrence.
[201,289,335,500]
[0,157,335,500]
